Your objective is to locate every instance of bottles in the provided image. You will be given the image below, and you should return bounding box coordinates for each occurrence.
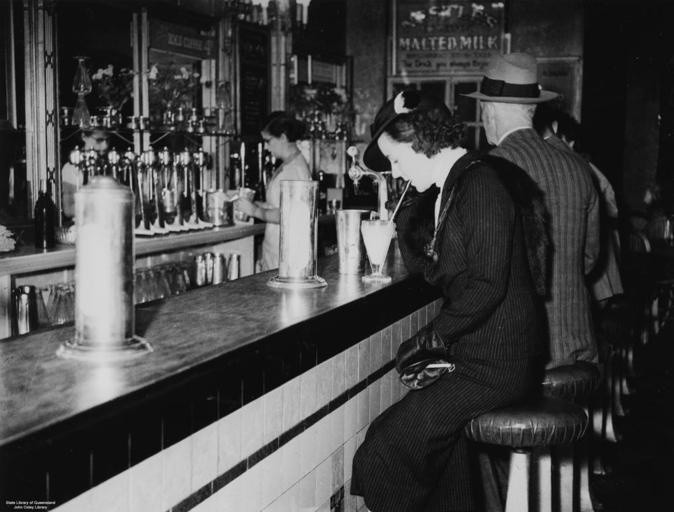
[35,189,55,249]
[194,251,239,287]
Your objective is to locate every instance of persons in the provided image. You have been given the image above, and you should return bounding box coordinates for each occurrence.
[533,101,626,309]
[233,109,314,275]
[59,120,113,227]
[346,86,548,510]
[458,49,608,373]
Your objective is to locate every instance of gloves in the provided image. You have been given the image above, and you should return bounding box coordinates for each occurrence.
[391,324,451,370]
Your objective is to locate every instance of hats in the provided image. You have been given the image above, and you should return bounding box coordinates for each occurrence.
[458,51,560,106]
[362,91,454,173]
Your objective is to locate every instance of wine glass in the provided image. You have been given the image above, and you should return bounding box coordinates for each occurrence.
[361,220,396,284]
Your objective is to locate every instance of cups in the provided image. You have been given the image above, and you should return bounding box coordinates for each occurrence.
[17,264,187,341]
[335,209,362,274]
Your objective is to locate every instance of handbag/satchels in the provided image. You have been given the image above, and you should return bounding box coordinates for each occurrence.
[399,357,456,391]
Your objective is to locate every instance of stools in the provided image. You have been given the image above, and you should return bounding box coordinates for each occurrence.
[466,229,674,493]
